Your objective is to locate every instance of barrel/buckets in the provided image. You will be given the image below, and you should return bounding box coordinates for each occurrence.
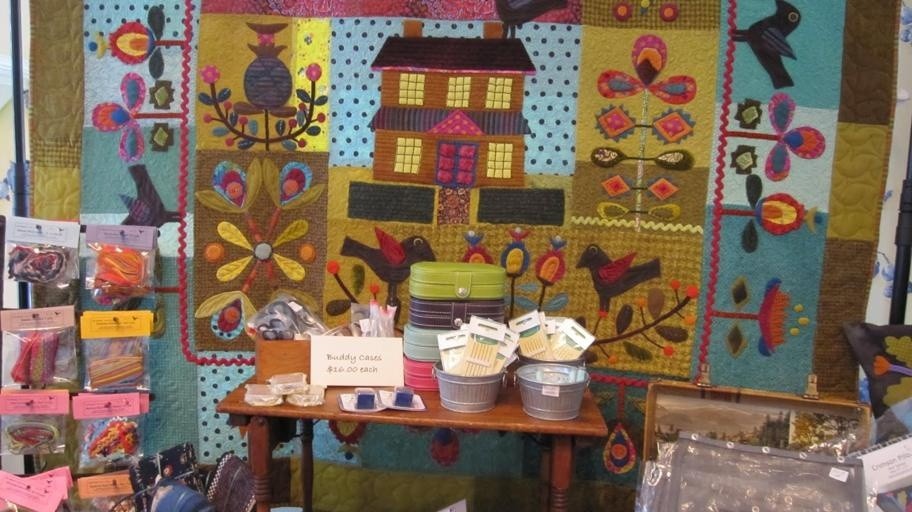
[431,359,506,413]
[513,364,592,420]
[515,348,596,367]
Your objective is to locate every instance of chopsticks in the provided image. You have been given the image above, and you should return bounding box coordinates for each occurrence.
[436,311,592,374]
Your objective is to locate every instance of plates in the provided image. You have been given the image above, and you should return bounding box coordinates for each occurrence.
[336,391,386,413]
[378,389,427,412]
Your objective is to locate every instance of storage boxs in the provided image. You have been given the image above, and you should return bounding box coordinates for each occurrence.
[252,337,310,384]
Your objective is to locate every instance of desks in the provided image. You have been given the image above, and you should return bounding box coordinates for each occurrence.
[215,341,608,511]
